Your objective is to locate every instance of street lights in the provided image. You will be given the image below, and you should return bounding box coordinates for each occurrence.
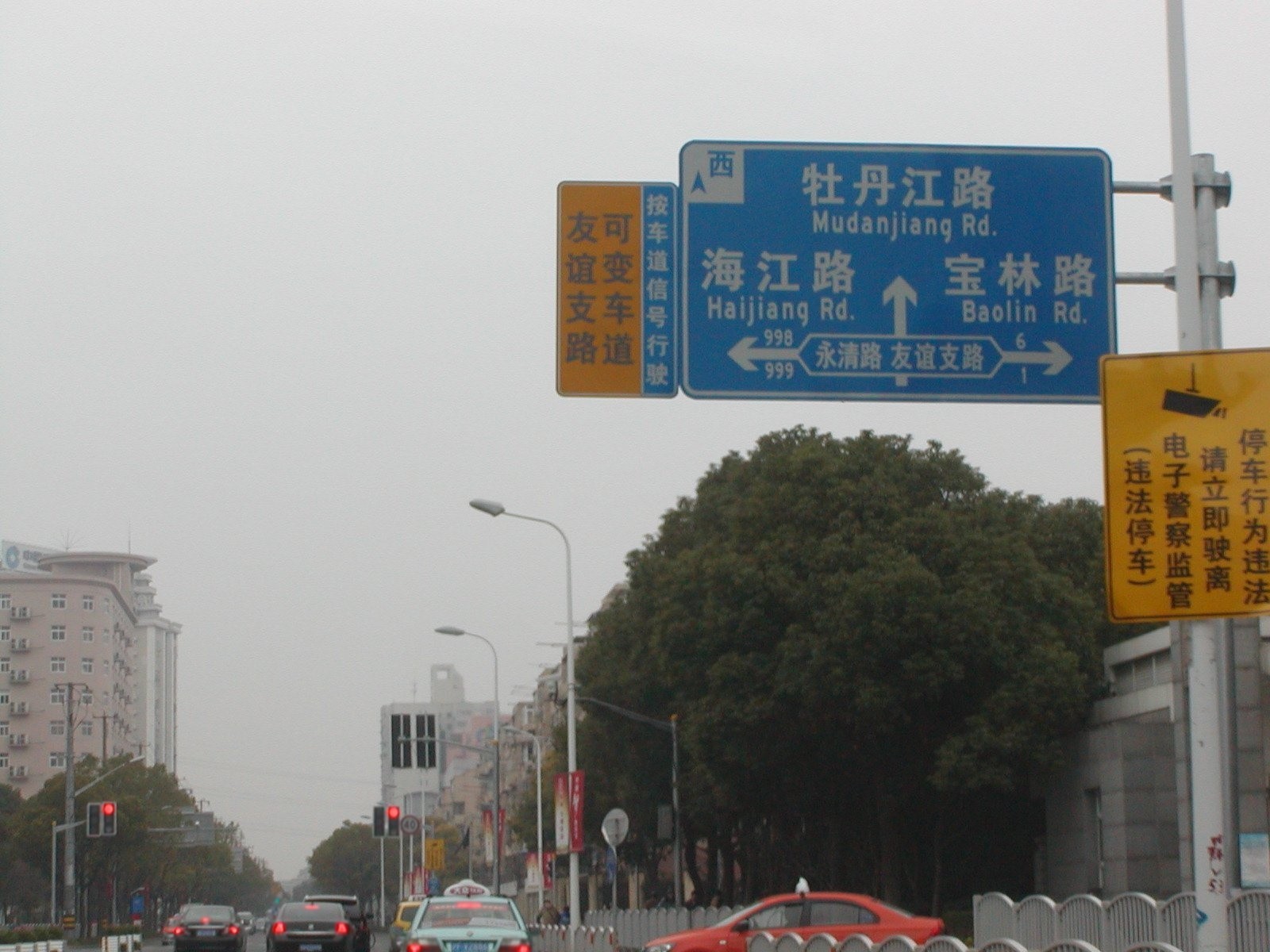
[397,735,501,896]
[502,724,543,911]
[63,755,145,939]
[469,498,581,929]
[435,627,500,899]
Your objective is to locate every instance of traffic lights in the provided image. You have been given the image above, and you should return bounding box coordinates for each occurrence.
[387,805,400,837]
[101,801,117,836]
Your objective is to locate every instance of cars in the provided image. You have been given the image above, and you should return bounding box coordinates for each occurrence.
[236,912,268,936]
[403,896,532,952]
[649,892,945,952]
[390,901,422,952]
[161,907,249,952]
[266,903,355,952]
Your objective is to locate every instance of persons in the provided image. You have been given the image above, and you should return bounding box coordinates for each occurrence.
[644,887,755,911]
[537,899,570,925]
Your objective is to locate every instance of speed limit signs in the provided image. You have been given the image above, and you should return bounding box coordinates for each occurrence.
[401,815,419,834]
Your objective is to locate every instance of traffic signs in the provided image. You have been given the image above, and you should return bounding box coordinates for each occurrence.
[680,139,1117,405]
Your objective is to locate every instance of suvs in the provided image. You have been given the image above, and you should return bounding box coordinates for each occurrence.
[303,893,373,952]
